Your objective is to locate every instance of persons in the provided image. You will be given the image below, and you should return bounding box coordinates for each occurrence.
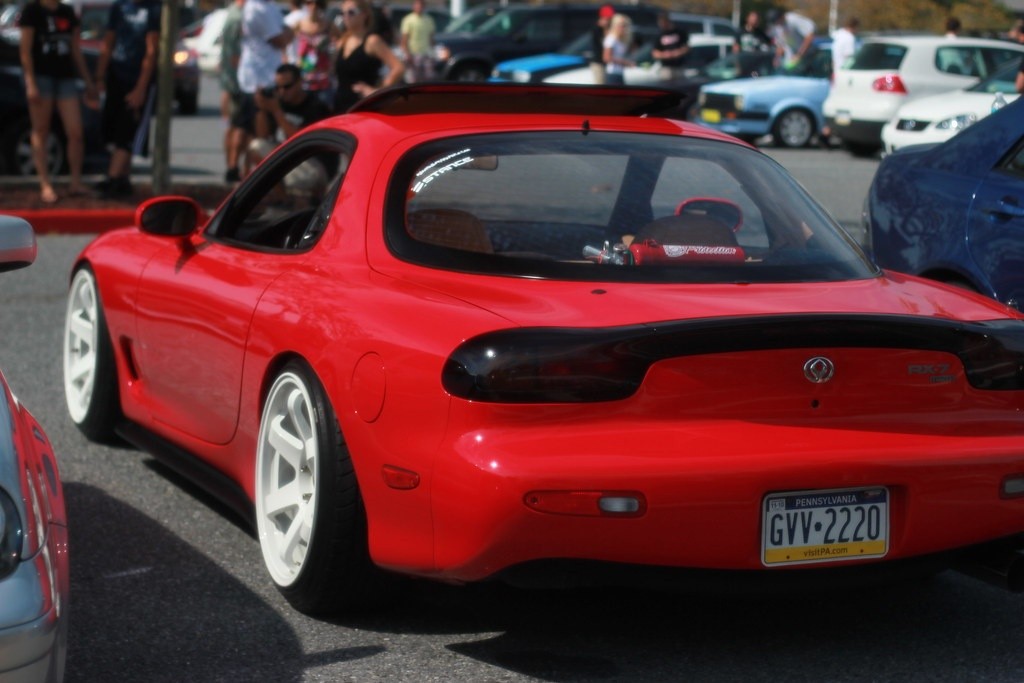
[590,7,637,85]
[943,16,964,38]
[1011,22,1024,90]
[821,18,860,145]
[219,0,437,206]
[732,9,818,74]
[16,0,92,203]
[652,10,688,78]
[95,0,163,198]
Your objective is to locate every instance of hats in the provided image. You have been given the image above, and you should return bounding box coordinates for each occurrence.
[601,6,615,18]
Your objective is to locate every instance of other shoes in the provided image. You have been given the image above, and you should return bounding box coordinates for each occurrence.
[94,175,132,197]
[67,183,92,195]
[226,165,240,182]
[40,185,58,204]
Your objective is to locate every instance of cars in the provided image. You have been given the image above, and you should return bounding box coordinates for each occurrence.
[178,1,229,74]
[0,0,199,115]
[61,79,1024,614]
[430,0,833,149]
[880,53,1024,154]
[0,213,70,683]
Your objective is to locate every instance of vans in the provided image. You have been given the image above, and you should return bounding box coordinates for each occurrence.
[820,32,1024,158]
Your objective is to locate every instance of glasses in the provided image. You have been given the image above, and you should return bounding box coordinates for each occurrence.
[274,81,294,90]
[340,10,360,15]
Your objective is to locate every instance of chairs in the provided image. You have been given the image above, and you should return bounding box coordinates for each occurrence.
[405,209,494,255]
[629,215,740,258]
